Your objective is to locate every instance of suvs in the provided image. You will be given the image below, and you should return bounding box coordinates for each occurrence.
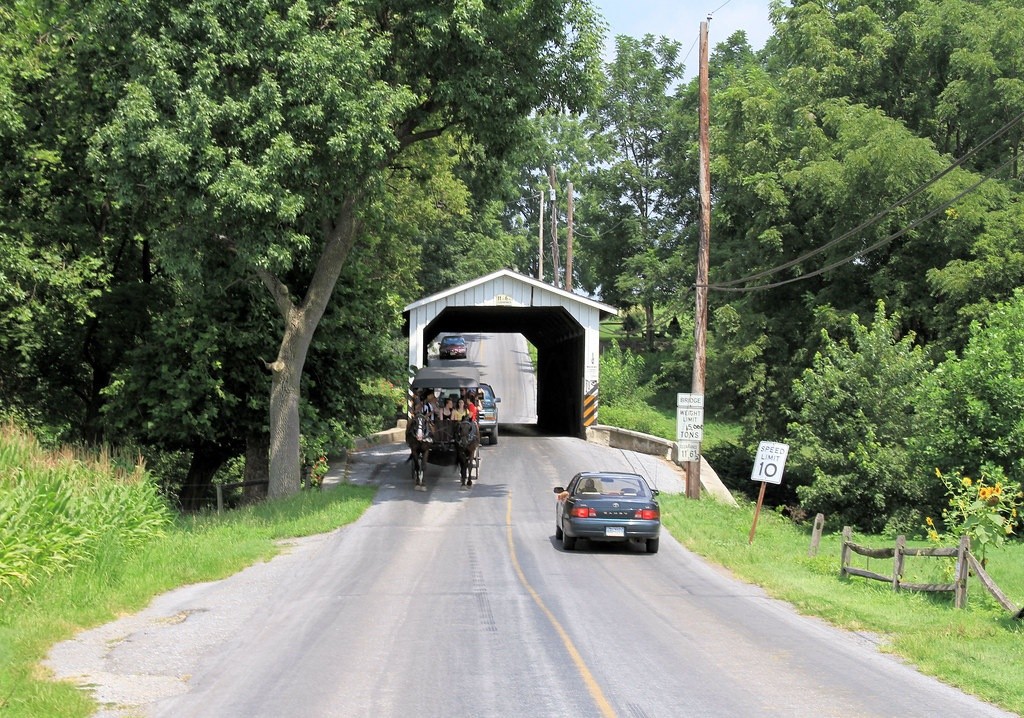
[466,382,502,446]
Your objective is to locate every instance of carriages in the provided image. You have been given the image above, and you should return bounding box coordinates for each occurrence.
[405,367,484,490]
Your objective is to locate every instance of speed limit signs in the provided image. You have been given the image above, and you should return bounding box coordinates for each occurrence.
[750,441,790,486]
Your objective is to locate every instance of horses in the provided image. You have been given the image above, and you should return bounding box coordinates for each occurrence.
[405,404,480,492]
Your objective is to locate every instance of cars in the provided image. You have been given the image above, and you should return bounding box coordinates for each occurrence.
[553,471,661,553]
[438,336,469,360]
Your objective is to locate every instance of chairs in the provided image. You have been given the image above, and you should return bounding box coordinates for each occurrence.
[581,480,597,491]
[621,488,636,493]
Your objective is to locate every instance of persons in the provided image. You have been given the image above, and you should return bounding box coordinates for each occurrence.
[581,480,597,493]
[411,388,483,421]
